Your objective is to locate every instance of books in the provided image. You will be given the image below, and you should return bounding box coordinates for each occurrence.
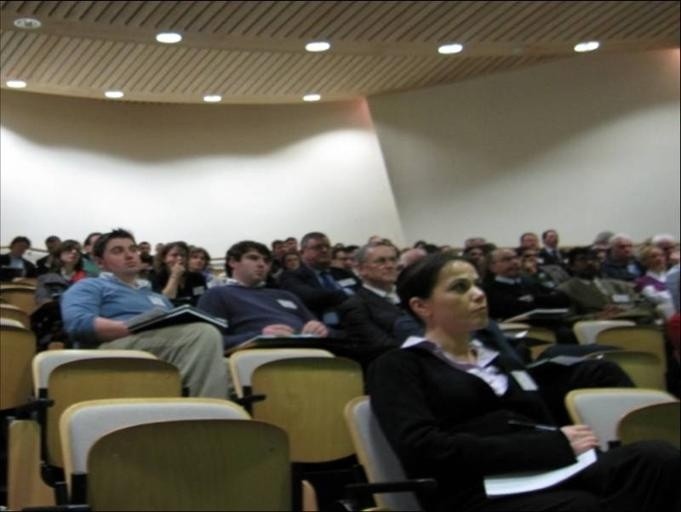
[127,303,228,329]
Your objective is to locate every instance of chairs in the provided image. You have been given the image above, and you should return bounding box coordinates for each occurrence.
[0,280,681,511]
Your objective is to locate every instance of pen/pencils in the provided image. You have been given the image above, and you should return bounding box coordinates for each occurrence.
[507,419,558,431]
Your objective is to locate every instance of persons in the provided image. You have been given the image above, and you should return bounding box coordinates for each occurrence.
[61,228,236,401]
[369,252,680,512]
[486,227,680,397]
[196,232,527,372]
[0,226,213,306]
